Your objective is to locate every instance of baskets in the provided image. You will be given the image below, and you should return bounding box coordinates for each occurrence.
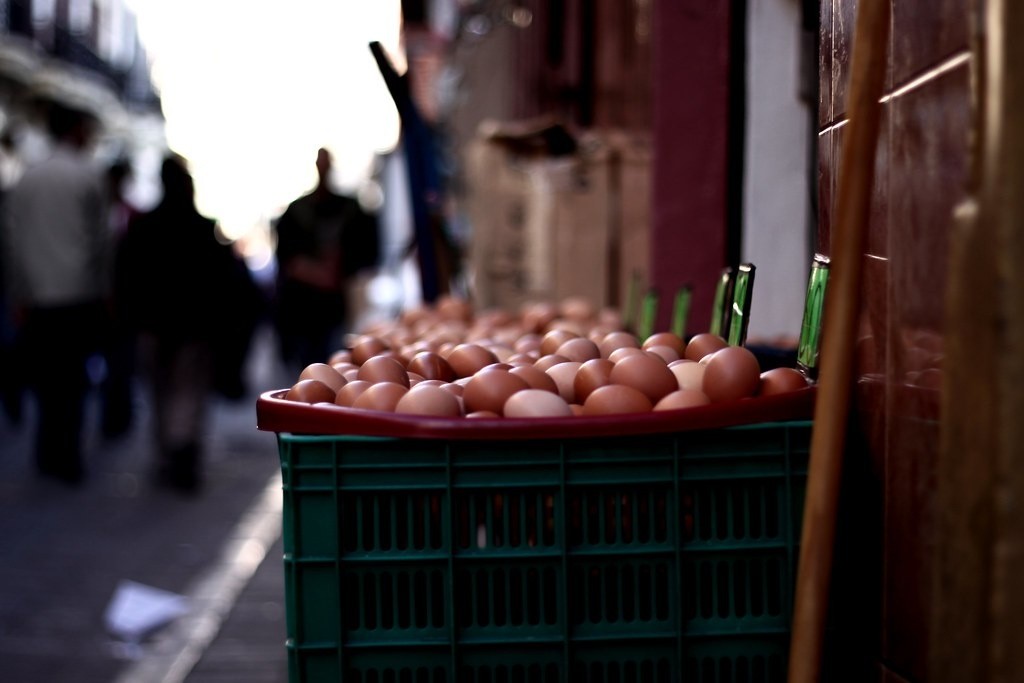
[257,385,869,683]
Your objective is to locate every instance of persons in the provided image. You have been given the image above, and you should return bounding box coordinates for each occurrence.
[272,145,386,382]
[0,104,261,505]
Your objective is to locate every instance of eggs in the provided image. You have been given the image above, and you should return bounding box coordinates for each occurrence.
[279,294,811,420]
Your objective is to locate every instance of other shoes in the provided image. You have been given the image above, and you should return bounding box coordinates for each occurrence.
[148,438,204,494]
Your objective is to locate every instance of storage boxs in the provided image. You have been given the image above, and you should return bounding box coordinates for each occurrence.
[255,391,862,683]
